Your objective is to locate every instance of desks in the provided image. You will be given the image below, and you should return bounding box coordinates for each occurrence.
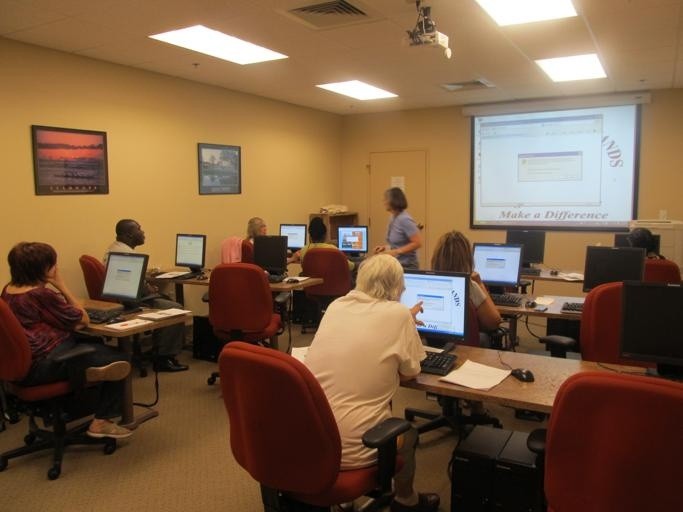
[515,263,586,286]
[145,262,324,351]
[477,289,582,357]
[386,333,659,510]
[71,298,194,456]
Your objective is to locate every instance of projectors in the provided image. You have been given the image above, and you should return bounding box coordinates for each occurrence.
[408,30,449,49]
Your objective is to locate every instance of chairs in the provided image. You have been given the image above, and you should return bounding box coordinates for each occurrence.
[220,239,255,265]
[521,370,683,512]
[76,253,154,378]
[206,263,284,387]
[404,298,501,437]
[643,258,682,287]
[215,337,419,511]
[575,280,665,373]
[0,297,116,481]
[300,246,351,336]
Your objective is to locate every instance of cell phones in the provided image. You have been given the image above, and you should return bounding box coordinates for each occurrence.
[535,307,548,312]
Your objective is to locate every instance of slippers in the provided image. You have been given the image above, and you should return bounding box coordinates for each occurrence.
[85,361,130,382]
[86,423,131,437]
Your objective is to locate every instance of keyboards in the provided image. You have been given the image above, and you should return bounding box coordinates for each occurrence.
[520,268,541,276]
[489,294,522,307]
[420,353,458,376]
[88,312,109,324]
[560,302,584,313]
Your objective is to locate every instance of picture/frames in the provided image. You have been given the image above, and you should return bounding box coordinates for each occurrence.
[195,142,242,195]
[29,124,109,197]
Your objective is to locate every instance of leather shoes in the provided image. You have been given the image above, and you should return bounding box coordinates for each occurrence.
[390,493,440,512]
[154,358,190,373]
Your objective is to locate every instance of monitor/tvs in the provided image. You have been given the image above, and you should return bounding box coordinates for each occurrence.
[615,233,660,254]
[175,234,206,275]
[337,226,368,257]
[101,252,149,313]
[254,235,287,275]
[619,279,683,382]
[506,229,545,266]
[280,224,307,253]
[583,245,646,293]
[399,269,470,354]
[472,243,525,294]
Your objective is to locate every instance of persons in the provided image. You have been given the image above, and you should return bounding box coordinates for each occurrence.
[627,227,667,261]
[430,229,502,426]
[244,217,300,264]
[0,240,134,439]
[303,253,440,512]
[372,186,422,271]
[103,219,190,372]
[300,216,356,274]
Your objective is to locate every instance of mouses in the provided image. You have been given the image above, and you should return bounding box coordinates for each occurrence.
[512,368,534,382]
[551,271,558,276]
[116,317,126,322]
[525,302,536,308]
[288,279,299,283]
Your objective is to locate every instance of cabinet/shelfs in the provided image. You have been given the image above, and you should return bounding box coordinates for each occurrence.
[308,210,359,250]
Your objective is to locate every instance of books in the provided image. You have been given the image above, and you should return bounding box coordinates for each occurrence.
[104,319,154,330]
[137,306,193,321]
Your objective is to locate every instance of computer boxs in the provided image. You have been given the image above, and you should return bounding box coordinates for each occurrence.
[292,289,305,324]
[498,430,547,512]
[450,425,513,512]
[205,318,225,363]
[41,343,122,427]
[515,408,546,422]
[192,315,208,360]
[546,318,580,358]
[305,296,319,324]
